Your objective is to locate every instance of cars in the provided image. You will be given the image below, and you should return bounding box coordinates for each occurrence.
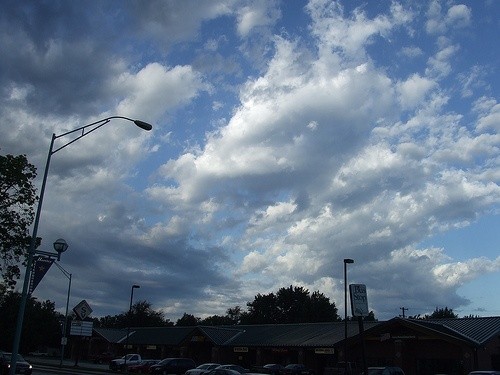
[0,351,34,375]
[360,366,405,375]
[261,363,307,375]
[128,361,154,375]
[185,363,251,375]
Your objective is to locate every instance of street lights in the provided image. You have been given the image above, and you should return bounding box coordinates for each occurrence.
[343,258,354,361]
[124,284,140,366]
[44,256,73,375]
[9,115,152,375]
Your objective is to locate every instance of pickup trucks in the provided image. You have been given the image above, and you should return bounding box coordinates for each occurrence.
[110,353,161,372]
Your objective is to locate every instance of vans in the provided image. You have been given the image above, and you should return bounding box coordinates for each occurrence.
[148,357,193,375]
